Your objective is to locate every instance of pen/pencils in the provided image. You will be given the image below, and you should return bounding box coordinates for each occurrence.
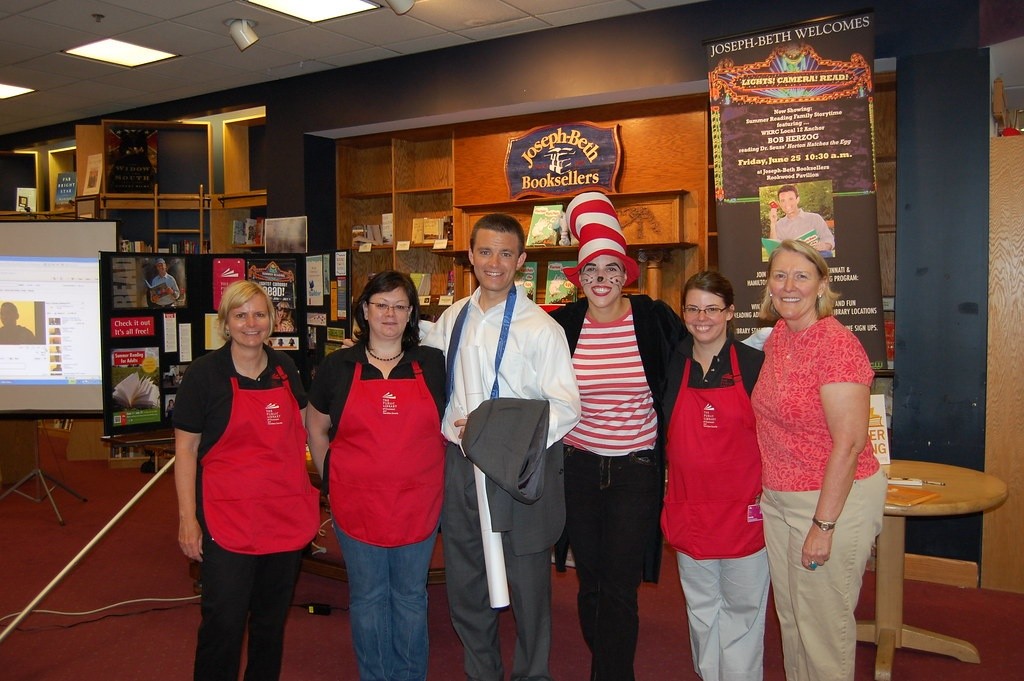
[923,480,946,485]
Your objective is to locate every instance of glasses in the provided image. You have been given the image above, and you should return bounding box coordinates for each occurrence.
[682,303,728,318]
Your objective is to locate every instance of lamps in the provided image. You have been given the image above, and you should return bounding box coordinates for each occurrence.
[386,0,416,15]
[228,18,259,53]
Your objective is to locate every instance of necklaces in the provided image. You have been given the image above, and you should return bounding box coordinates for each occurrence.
[785,316,816,359]
[366,347,404,361]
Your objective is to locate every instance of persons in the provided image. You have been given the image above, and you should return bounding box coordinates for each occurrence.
[662,268,766,681]
[174,280,321,681]
[152,257,181,306]
[304,270,450,681]
[341,213,581,681]
[769,185,834,251]
[752,238,888,681]
[545,192,693,681]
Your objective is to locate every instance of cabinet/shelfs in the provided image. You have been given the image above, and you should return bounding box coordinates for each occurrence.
[334,71,707,376]
[74,111,266,255]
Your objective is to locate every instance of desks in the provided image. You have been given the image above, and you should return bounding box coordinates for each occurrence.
[855,459,1008,681]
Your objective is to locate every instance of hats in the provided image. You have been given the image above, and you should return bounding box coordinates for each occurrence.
[562,191,640,289]
[155,258,165,264]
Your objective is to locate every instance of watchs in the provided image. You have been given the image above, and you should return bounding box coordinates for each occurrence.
[811,514,836,531]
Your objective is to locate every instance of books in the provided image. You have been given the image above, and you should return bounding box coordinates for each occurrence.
[349,204,597,310]
[762,229,821,258]
[112,372,159,407]
[144,280,170,299]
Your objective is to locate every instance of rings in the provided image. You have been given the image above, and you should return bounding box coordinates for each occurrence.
[808,561,818,570]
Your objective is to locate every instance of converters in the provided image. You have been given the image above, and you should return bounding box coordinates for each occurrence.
[308,603,331,616]
